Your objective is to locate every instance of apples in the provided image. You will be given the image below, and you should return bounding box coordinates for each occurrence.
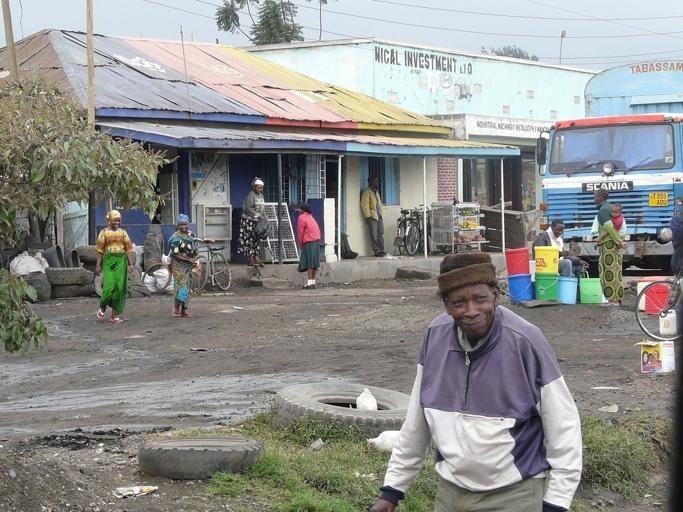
[460,210,477,216]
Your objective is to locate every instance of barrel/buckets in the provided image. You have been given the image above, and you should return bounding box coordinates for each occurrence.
[505,246,603,304]
[637,281,676,336]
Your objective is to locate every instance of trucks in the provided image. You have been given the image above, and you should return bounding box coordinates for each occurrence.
[535,58,683,276]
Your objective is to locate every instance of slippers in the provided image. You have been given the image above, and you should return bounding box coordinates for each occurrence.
[96,307,105,320]
[109,316,123,324]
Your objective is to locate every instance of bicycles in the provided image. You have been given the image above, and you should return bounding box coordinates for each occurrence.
[188,238,232,291]
[394,203,448,256]
[634,270,683,343]
[141,229,209,298]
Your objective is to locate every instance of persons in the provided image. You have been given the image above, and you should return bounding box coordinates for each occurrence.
[670,209,683,278]
[360,175,389,258]
[238,178,268,267]
[370,250,586,512]
[92,209,134,323]
[529,217,589,278]
[591,187,629,307]
[642,350,661,372]
[166,213,200,318]
[294,203,321,289]
[593,202,626,245]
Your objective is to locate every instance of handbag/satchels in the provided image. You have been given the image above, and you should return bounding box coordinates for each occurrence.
[255,217,270,239]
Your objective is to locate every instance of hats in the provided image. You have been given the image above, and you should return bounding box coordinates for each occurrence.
[437,252,497,294]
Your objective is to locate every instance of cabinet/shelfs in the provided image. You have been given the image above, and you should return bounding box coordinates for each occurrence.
[197,202,233,242]
[427,200,491,253]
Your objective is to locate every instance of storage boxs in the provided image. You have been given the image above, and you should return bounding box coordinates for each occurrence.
[633,340,675,374]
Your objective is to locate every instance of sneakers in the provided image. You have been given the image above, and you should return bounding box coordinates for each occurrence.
[302,283,316,289]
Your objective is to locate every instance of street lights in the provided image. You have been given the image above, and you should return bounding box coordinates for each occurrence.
[557,28,565,65]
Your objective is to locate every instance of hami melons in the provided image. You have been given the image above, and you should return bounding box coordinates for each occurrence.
[454,235,485,243]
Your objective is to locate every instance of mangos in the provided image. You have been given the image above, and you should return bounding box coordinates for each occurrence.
[463,220,480,228]
